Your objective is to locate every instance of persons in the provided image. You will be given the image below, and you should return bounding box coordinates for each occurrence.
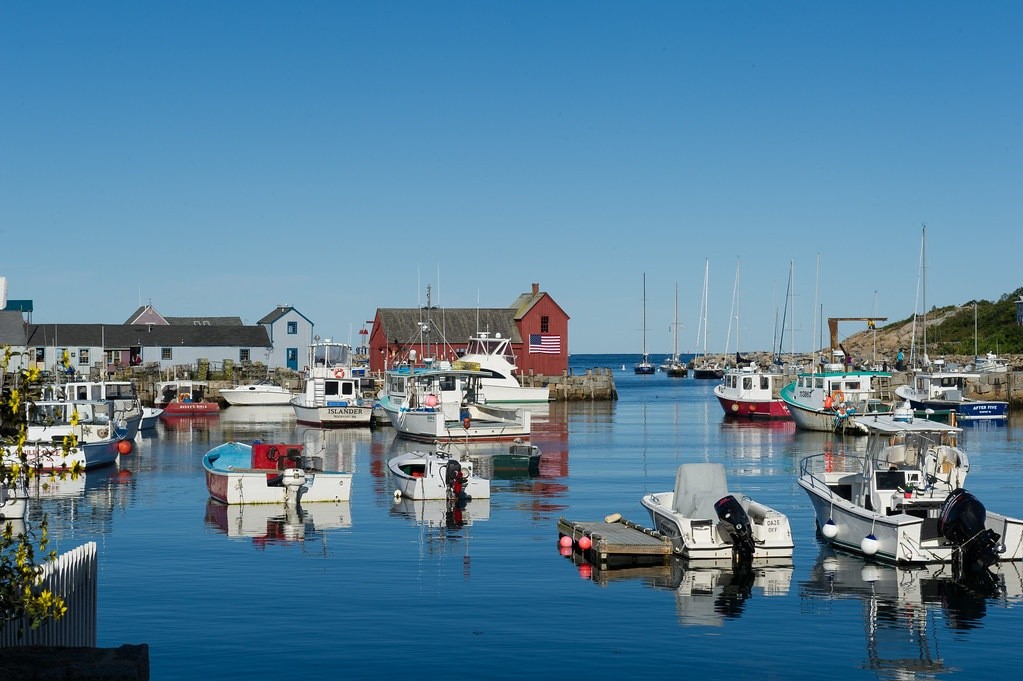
[844,353,853,366]
[897,348,904,371]
[163,388,203,402]
[129,354,142,366]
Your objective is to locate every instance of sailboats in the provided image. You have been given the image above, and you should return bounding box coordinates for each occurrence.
[633,273,656,374]
[659,281,689,376]
[693,259,727,379]
[774,223,946,372]
[718,260,762,373]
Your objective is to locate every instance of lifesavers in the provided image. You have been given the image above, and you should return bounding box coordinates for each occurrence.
[114,403,117,412]
[335,368,344,378]
[124,401,133,411]
[346,398,353,406]
[461,468,469,478]
[98,429,105,438]
[178,394,189,402]
[832,390,844,403]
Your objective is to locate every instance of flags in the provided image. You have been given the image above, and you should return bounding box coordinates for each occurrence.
[529,334,560,354]
[736,352,753,364]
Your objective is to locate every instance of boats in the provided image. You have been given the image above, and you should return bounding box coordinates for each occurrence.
[796,431,1023,567]
[200,434,353,505]
[894,373,1009,421]
[714,362,791,417]
[0,386,130,473]
[0,467,28,519]
[288,334,373,426]
[386,450,491,500]
[58,326,145,441]
[640,463,795,560]
[218,341,295,406]
[377,283,550,443]
[153,379,222,412]
[969,303,1008,372]
[138,382,165,430]
[362,368,392,426]
[778,372,950,432]
